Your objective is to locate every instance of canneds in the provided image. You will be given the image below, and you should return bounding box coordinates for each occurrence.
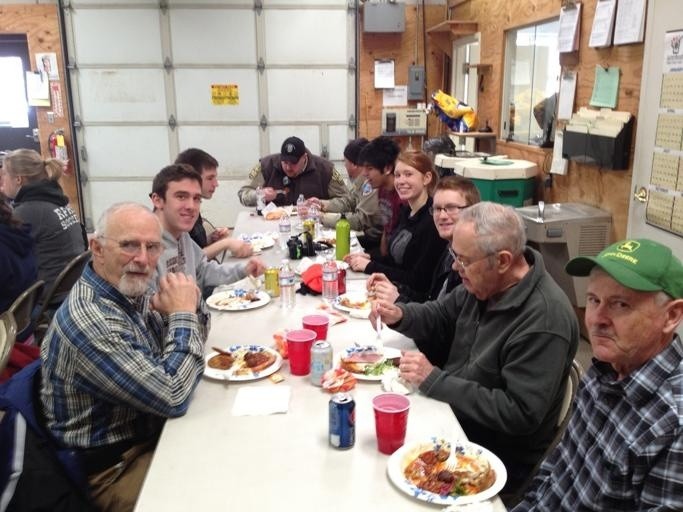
[263,265,280,298]
[337,269,347,294]
[329,393,356,450]
[310,339,333,387]
[303,219,315,240]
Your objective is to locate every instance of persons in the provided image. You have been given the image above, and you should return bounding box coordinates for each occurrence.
[510,238,683,512]
[175,149,229,250]
[369,201,580,504]
[1,148,87,310]
[40,201,211,512]
[0,193,38,343]
[365,175,481,372]
[305,137,380,233]
[343,149,439,286]
[149,164,265,298]
[238,138,351,214]
[358,136,410,255]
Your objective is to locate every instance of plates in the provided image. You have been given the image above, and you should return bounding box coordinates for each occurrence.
[332,289,372,313]
[239,231,274,250]
[339,345,410,381]
[203,289,273,312]
[202,344,282,383]
[385,441,506,505]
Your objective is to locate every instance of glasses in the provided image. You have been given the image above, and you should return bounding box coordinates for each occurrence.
[450,249,499,267]
[429,204,470,216]
[98,234,162,257]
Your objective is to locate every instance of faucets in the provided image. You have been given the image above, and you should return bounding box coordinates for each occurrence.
[537,200,546,217]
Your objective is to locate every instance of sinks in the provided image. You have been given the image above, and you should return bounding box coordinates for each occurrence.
[515,200,610,246]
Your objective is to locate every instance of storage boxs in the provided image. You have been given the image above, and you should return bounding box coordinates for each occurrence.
[453,157,541,208]
[434,148,492,178]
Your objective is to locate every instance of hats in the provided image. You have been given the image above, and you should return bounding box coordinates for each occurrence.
[343,137,369,168]
[280,136,306,165]
[563,238,682,302]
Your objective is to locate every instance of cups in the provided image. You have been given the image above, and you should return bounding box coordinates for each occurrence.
[285,328,313,376]
[373,392,411,454]
[302,314,329,340]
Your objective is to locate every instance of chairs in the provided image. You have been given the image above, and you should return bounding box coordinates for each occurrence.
[0,248,102,511]
[507,356,583,511]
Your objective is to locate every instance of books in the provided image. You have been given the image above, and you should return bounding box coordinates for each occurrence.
[549,1,647,176]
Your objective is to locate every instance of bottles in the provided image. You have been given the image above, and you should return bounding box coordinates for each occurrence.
[255,182,265,212]
[279,213,292,250]
[278,257,296,309]
[295,194,308,224]
[320,254,339,303]
[309,202,319,237]
